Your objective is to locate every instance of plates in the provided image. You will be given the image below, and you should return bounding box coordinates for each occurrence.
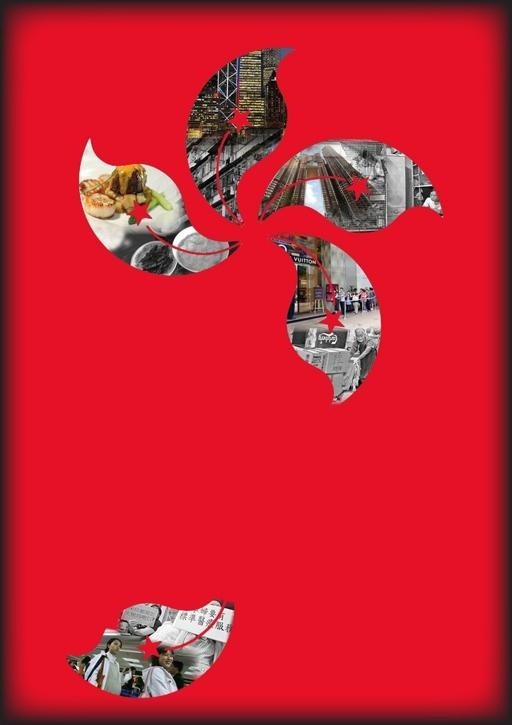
[172,225,230,273]
[130,240,178,277]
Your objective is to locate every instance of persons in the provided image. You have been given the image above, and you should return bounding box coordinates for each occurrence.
[68,655,185,697]
[143,599,225,689]
[348,327,377,382]
[422,190,443,218]
[332,286,377,316]
[85,638,123,697]
[139,645,178,699]
[122,605,162,638]
